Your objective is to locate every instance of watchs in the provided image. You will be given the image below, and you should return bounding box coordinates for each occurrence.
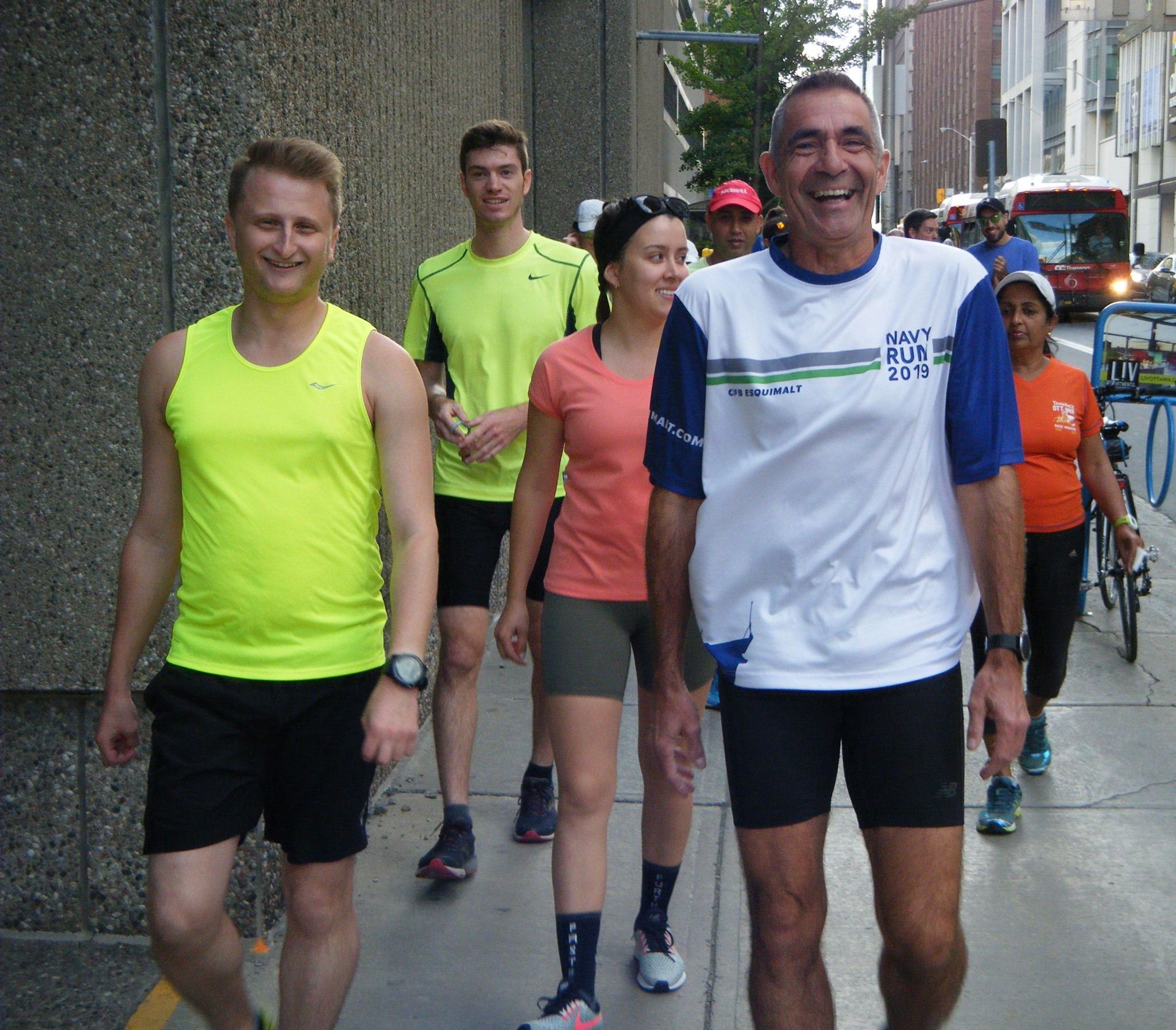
[1112,513,1138,530]
[985,630,1031,661]
[381,652,429,699]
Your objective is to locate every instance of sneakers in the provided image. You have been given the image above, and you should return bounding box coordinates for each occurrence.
[414,820,478,881]
[631,918,687,992]
[516,979,602,1030]
[975,776,1022,833]
[513,775,557,843]
[1019,688,1051,774]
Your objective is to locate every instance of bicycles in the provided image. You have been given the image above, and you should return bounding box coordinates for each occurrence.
[1086,378,1162,663]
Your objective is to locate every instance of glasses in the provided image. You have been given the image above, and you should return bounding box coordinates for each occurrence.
[579,230,594,238]
[976,213,1004,226]
[625,194,690,219]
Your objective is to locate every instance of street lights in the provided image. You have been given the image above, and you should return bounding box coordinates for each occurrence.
[921,160,948,201]
[939,127,974,196]
[1055,66,1101,177]
[1009,100,1044,172]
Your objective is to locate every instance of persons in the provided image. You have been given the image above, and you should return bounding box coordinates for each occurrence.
[971,271,1144,836]
[494,195,716,1030]
[560,178,1042,709]
[641,72,1030,1030]
[95,135,438,1030]
[404,118,611,885]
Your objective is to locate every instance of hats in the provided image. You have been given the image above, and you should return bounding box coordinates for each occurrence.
[975,197,1005,215]
[575,199,604,232]
[707,179,762,218]
[995,270,1055,312]
[686,239,699,263]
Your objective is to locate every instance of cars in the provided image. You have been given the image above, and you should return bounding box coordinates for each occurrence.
[1130,251,1172,298]
[1144,253,1175,304]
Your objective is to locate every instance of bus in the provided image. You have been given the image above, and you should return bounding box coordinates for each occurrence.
[940,172,1133,320]
